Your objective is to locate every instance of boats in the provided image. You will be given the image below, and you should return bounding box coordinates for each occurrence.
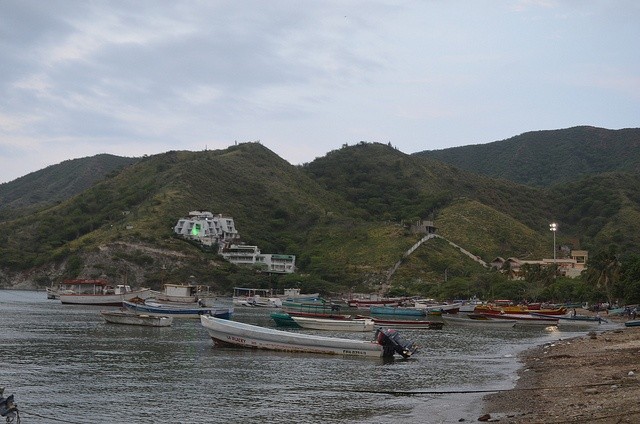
[60,288,151,304]
[247,295,282,307]
[141,284,205,308]
[232,287,273,307]
[352,315,444,330]
[100,311,173,327]
[200,314,396,358]
[458,300,477,311]
[271,306,351,328]
[414,302,462,314]
[46,280,110,298]
[291,316,375,332]
[119,298,234,319]
[468,305,570,324]
[558,316,625,332]
[347,300,406,309]
[368,305,442,320]
[441,315,516,327]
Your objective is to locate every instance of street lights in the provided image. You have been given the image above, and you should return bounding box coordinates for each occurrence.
[550,223,557,267]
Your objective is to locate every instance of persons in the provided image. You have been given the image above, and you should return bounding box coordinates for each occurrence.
[627,304,636,319]
[573,308,576,317]
